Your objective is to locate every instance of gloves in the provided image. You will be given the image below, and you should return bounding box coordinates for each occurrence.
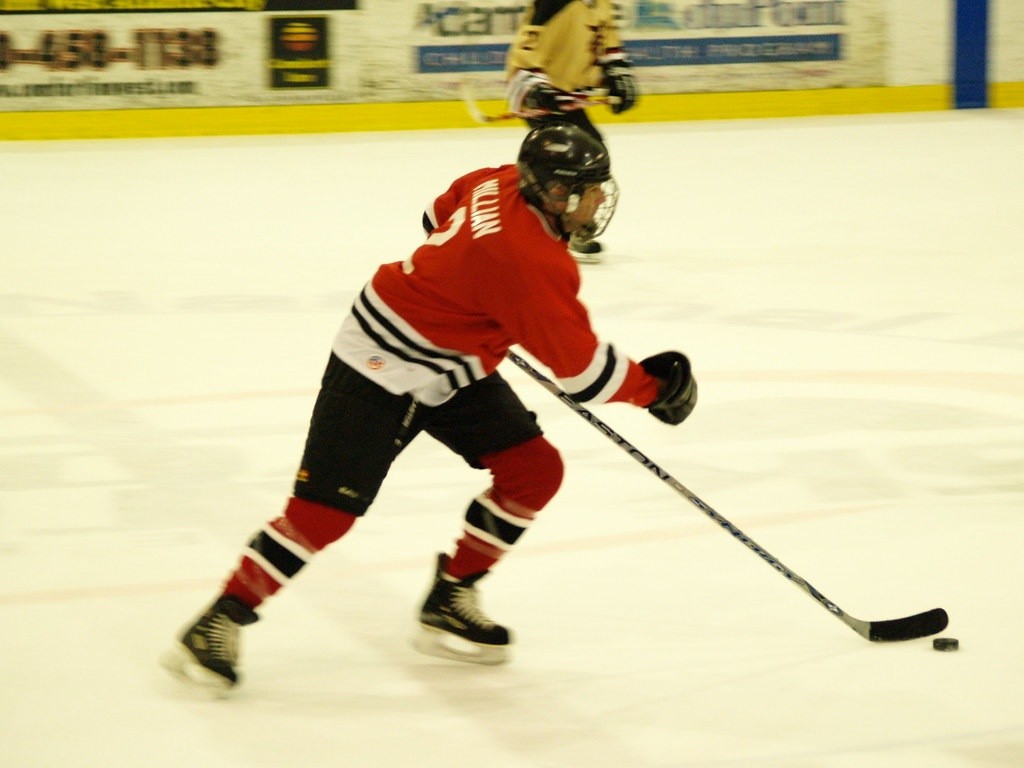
[523,84,581,112]
[638,351,697,424]
[601,59,635,113]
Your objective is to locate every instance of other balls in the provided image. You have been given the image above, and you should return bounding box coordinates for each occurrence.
[932,638,959,652]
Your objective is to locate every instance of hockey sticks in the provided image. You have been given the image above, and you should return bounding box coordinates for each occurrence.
[458,81,624,127]
[504,348,948,643]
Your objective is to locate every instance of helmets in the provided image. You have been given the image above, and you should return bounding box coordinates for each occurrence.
[515,125,613,241]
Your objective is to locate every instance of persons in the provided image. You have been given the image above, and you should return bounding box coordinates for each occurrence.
[159,119,696,701]
[506,0,640,264]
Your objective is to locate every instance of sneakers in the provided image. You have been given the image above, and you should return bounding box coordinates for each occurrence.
[565,219,601,262]
[419,553,509,664]
[170,593,258,688]
